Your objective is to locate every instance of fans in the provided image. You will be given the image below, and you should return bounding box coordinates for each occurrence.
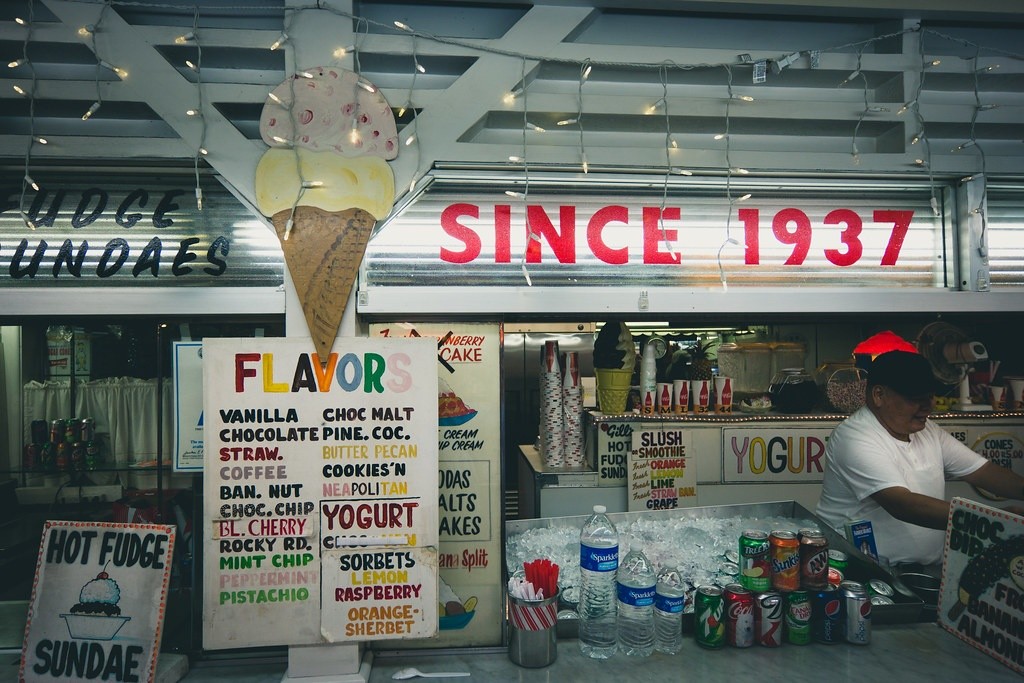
[915,320,994,411]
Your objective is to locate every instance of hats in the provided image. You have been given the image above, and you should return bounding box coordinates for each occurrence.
[866,350,949,396]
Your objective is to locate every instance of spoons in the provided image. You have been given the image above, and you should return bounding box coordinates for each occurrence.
[392,667,471,680]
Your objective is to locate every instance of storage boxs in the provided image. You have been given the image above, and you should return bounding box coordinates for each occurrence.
[769,343,808,383]
[715,342,772,391]
[504,500,925,638]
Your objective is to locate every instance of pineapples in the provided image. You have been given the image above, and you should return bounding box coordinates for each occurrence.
[685,340,716,380]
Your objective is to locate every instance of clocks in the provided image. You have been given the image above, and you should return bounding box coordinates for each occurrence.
[647,337,668,360]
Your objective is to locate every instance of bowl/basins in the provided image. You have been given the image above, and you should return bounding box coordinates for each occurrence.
[741,405,774,414]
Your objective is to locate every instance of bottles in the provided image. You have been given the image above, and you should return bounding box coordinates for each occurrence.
[653,559,682,655]
[616,544,654,658]
[579,505,617,660]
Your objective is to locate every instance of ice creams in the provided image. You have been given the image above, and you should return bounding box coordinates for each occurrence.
[593,319,636,415]
[254,67,399,366]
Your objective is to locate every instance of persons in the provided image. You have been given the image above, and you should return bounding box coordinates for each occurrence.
[816,348,1024,564]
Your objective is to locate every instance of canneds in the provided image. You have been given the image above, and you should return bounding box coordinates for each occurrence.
[556,586,581,620]
[685,529,895,645]
[23,417,99,471]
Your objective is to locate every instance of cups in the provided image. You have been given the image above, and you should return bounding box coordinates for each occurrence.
[508,586,559,669]
[1011,380,1024,403]
[640,343,734,416]
[534,340,585,469]
[986,386,1008,411]
